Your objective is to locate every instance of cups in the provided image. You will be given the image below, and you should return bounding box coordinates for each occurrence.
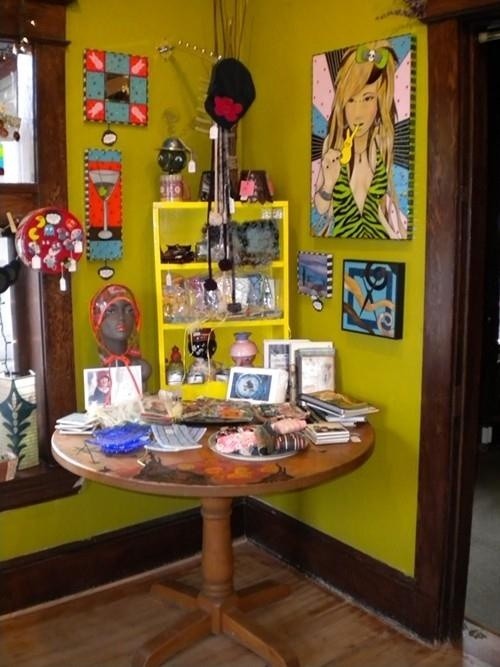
[160,173,182,201]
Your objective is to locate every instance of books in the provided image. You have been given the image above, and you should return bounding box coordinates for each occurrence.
[310,405,367,423]
[299,387,375,415]
[303,400,380,418]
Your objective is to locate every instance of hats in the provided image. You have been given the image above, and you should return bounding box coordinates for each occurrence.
[203,58,255,131]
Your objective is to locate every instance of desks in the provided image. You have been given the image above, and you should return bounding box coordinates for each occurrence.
[50,411,375,666]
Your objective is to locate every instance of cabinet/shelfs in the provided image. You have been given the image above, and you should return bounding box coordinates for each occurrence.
[151,199,291,400]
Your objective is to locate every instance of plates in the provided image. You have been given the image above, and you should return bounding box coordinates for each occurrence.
[93,420,208,455]
[306,422,350,445]
[207,428,298,459]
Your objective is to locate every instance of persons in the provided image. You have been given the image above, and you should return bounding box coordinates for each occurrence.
[90,283,154,384]
[88,368,111,403]
[312,39,404,238]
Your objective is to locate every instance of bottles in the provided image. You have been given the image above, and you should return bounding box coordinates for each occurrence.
[167,345,186,384]
[170,395,184,416]
[230,333,258,365]
